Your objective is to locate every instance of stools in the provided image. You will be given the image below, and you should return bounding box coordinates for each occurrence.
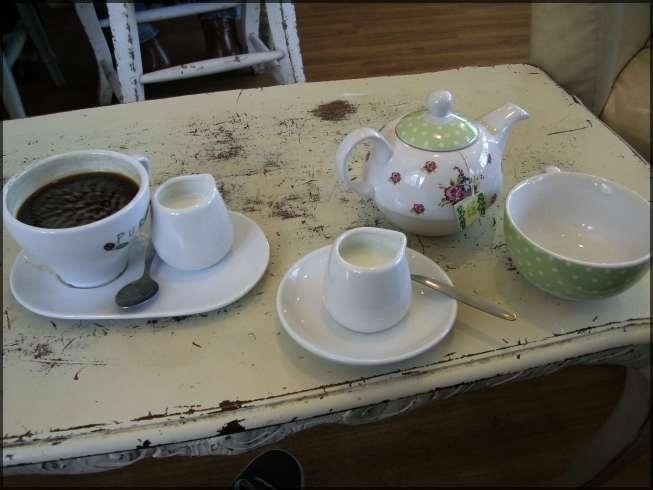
[74,1,308,105]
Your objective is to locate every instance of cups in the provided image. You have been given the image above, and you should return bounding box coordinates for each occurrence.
[504,163,652,302]
[153,173,233,273]
[328,225,409,336]
[1,150,151,287]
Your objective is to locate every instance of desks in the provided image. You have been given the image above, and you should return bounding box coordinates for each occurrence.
[0,61,653,489]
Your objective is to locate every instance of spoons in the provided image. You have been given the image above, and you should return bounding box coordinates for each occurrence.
[115,237,158,309]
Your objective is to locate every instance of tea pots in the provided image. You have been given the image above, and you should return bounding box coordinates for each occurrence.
[338,89,530,237]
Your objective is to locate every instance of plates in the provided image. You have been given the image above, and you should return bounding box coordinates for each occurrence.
[275,245,461,368]
[11,208,270,320]
